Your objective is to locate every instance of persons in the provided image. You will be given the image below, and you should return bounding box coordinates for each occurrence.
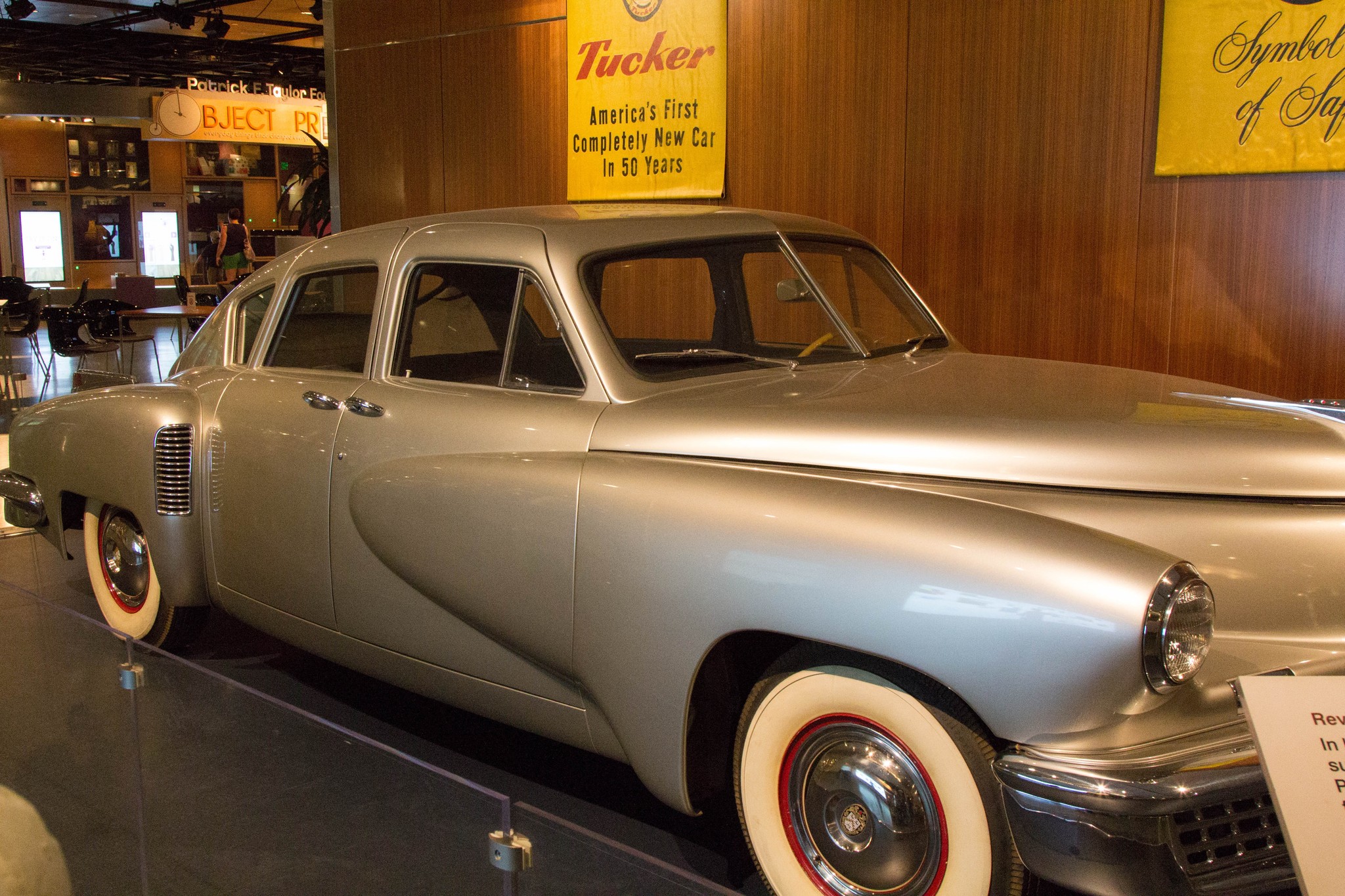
[194,219,225,285]
[216,208,248,281]
[75,208,116,258]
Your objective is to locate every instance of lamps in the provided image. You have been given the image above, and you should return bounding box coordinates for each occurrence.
[5,0,36,20]
[309,0,323,21]
[201,9,229,38]
[153,1,195,31]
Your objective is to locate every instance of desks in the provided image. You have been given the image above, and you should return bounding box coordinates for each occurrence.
[116,304,217,374]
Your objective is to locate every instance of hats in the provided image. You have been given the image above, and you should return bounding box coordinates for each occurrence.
[210,230,221,241]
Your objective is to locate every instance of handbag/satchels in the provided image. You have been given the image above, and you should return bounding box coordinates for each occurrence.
[244,225,256,262]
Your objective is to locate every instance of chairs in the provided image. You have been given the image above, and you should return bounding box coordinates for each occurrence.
[81,298,161,383]
[184,293,220,349]
[0,293,51,384]
[38,307,121,402]
[41,278,91,320]
[170,274,191,340]
[0,276,37,347]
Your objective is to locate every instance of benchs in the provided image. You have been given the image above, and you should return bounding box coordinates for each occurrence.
[401,339,669,387]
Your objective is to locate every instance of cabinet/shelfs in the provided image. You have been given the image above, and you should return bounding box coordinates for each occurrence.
[183,140,318,230]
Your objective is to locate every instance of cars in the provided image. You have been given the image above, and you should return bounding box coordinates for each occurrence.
[0,199,1344,896]
